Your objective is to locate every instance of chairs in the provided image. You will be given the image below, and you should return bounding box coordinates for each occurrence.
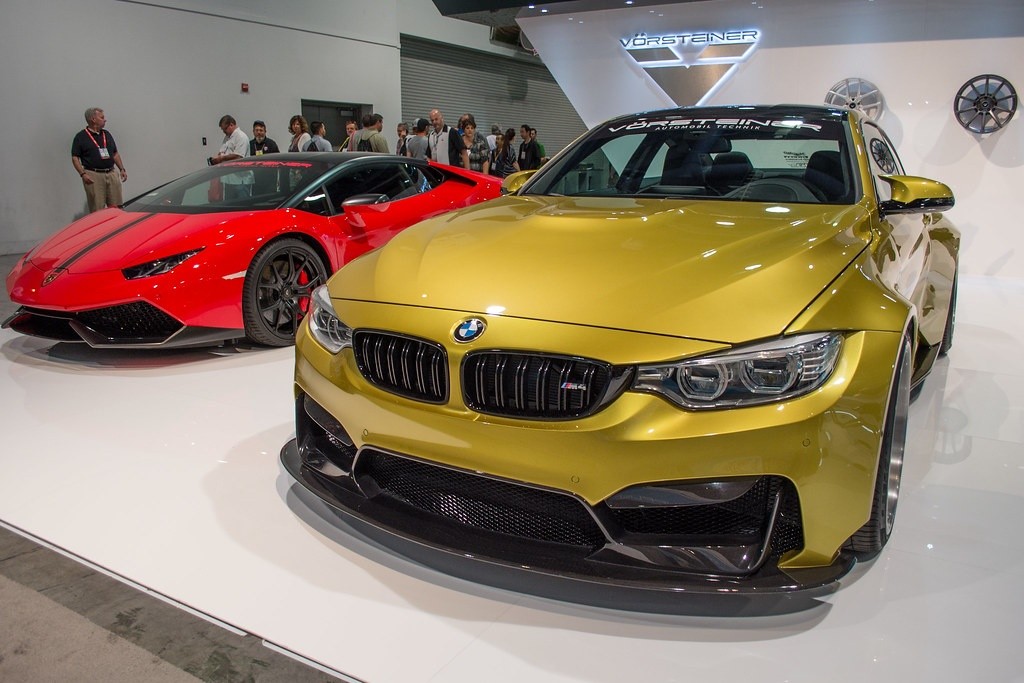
[661,144,705,186]
[804,151,846,199]
[707,152,753,192]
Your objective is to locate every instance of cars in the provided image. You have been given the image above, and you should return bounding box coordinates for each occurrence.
[280,103,962,597]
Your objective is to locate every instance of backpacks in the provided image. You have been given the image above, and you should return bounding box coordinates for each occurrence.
[357,132,378,151]
[307,138,318,151]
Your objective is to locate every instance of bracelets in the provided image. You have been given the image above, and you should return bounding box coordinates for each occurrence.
[80,172,86,178]
[120,168,126,172]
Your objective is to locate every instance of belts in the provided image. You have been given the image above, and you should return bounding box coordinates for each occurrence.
[84,167,113,174]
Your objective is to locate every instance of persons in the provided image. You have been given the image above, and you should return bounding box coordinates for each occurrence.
[336,121,358,152]
[395,108,546,178]
[346,114,390,155]
[287,115,311,194]
[70,107,128,213]
[302,122,333,152]
[210,114,256,200]
[249,120,282,196]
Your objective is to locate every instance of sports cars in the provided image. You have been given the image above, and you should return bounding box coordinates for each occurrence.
[5,151,505,351]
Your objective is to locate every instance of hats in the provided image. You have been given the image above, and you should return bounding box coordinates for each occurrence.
[417,118,433,126]
[412,118,420,127]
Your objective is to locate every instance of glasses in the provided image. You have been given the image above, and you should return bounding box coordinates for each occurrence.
[220,123,230,131]
